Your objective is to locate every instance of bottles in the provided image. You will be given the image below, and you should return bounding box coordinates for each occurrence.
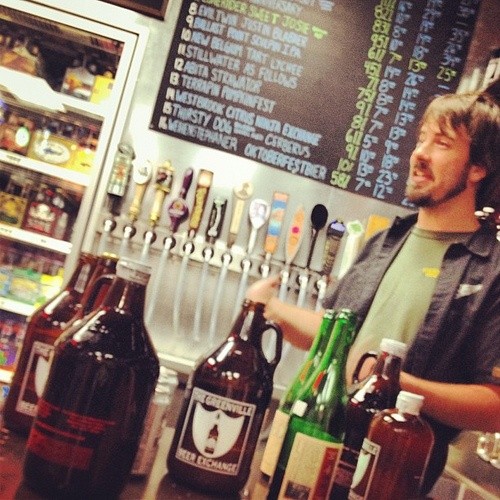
[266,308,359,500]
[166,297,283,497]
[3,252,100,432]
[20,255,159,500]
[259,309,341,485]
[62,253,120,334]
[346,390,435,500]
[328,338,409,500]
